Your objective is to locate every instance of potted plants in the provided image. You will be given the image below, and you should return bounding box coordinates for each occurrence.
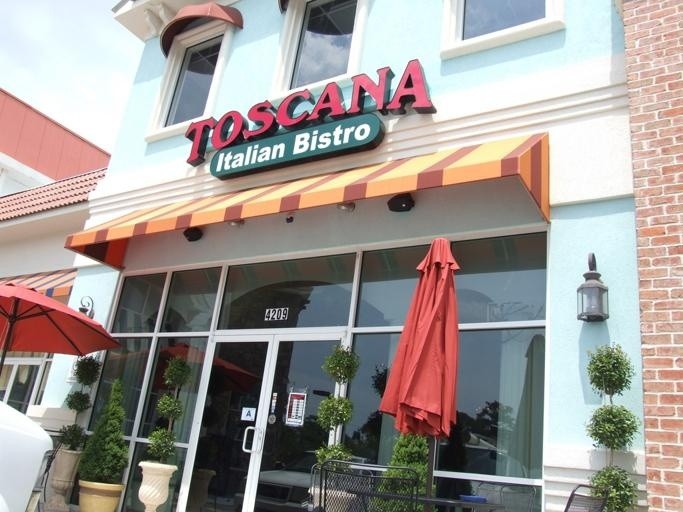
[135,354,193,512]
[183,401,219,511]
[77,375,130,512]
[303,343,363,512]
[38,353,103,512]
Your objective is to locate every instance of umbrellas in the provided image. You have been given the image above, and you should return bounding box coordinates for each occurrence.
[106,343,256,438]
[509,334,544,479]
[380,237,459,511]
[0,285,117,396]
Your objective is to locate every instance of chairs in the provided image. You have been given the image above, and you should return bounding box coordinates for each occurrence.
[563,483,609,512]
[307,458,538,512]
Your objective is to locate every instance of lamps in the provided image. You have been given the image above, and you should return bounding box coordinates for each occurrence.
[181,191,416,244]
[573,252,609,323]
[78,295,95,321]
